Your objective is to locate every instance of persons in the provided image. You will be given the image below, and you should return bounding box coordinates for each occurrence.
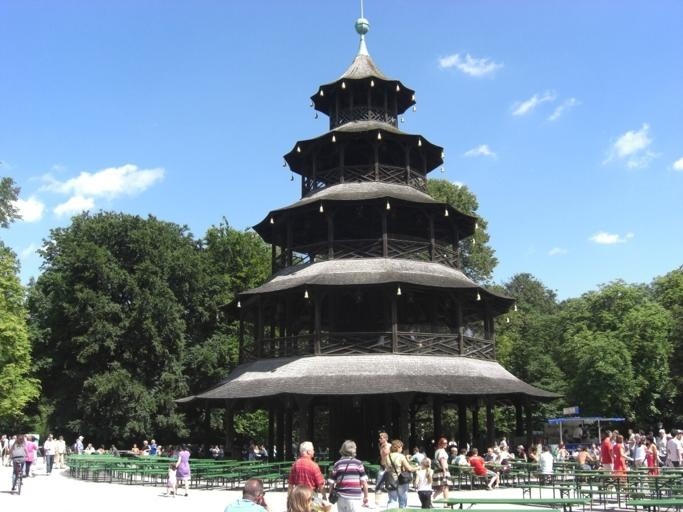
[131,439,224,458]
[164,463,177,495]
[74,436,119,457]
[225,478,268,511]
[1,432,66,490]
[175,443,191,496]
[248,443,287,461]
[287,427,682,511]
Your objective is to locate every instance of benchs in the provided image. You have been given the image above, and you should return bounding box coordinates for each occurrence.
[190,458,358,491]
[360,460,498,511]
[499,456,682,511]
[60,450,189,488]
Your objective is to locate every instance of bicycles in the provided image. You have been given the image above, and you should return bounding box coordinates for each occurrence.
[10,462,24,494]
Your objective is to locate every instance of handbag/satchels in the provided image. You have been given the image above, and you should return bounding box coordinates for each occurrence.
[431,459,442,472]
[328,481,337,504]
[398,471,411,485]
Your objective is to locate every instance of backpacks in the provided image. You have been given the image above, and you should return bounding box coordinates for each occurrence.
[385,470,398,490]
